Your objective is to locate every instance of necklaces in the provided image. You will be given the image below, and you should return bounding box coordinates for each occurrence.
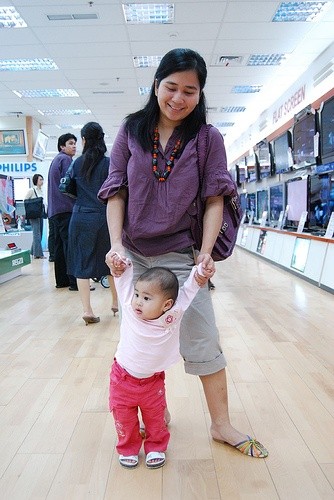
[152,127,183,182]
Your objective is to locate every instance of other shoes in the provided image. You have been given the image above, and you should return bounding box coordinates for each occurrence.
[49,256,56,262]
[68,288,77,290]
[56,282,70,288]
[34,256,47,259]
[30,254,36,259]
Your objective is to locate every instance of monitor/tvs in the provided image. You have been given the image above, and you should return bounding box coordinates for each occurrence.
[13,177,30,201]
[231,94,334,237]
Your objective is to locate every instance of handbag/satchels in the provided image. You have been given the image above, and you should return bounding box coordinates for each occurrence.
[197,124,243,261]
[57,155,83,199]
[24,188,45,220]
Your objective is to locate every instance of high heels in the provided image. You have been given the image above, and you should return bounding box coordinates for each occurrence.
[112,308,118,317]
[82,316,100,326]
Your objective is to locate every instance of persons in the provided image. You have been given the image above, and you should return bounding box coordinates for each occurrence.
[26,174,48,260]
[47,121,119,326]
[96,47,270,469]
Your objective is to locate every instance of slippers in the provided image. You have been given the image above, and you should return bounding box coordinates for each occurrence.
[213,426,268,458]
[138,424,168,439]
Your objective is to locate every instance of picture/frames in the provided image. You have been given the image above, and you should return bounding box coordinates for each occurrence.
[0,128,29,156]
[33,129,50,161]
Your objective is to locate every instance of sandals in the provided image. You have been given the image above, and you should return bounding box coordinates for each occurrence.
[145,452,166,469]
[119,453,139,468]
